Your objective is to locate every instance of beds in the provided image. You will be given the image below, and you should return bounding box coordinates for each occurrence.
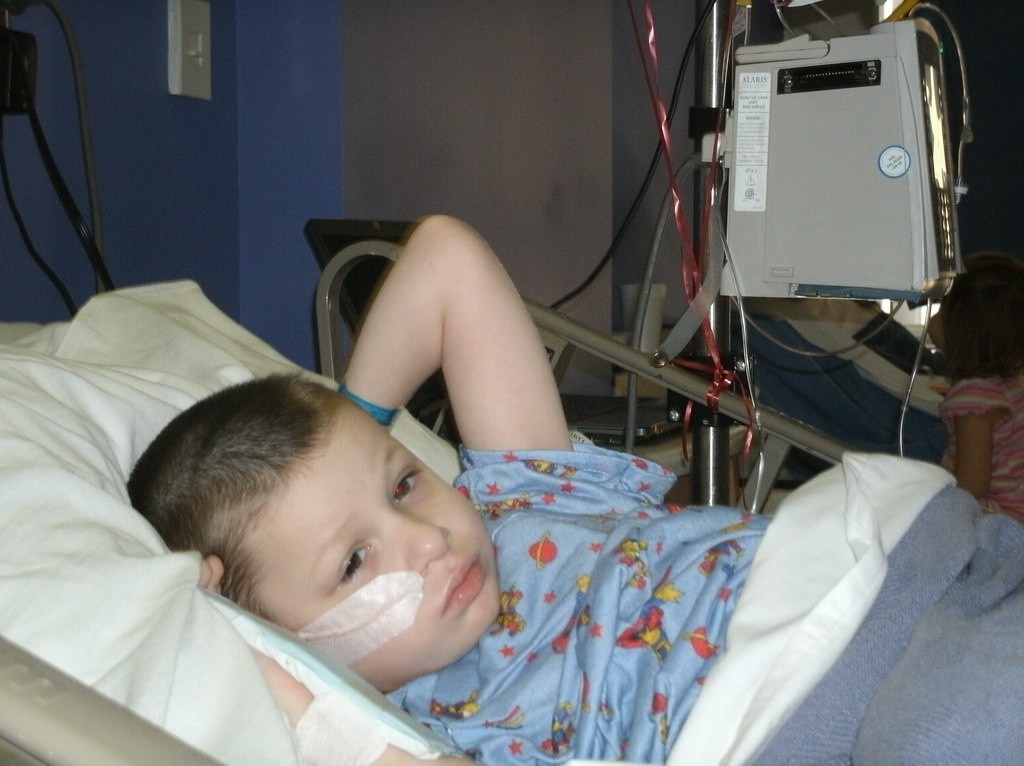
[723,299,949,516]
[2,236,862,766]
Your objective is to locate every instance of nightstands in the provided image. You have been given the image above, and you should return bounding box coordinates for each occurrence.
[590,422,751,508]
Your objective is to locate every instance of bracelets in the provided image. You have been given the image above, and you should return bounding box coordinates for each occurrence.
[337,378,397,425]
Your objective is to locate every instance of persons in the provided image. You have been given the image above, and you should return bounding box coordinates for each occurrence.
[936,253,1024,523]
[126,215,782,766]
